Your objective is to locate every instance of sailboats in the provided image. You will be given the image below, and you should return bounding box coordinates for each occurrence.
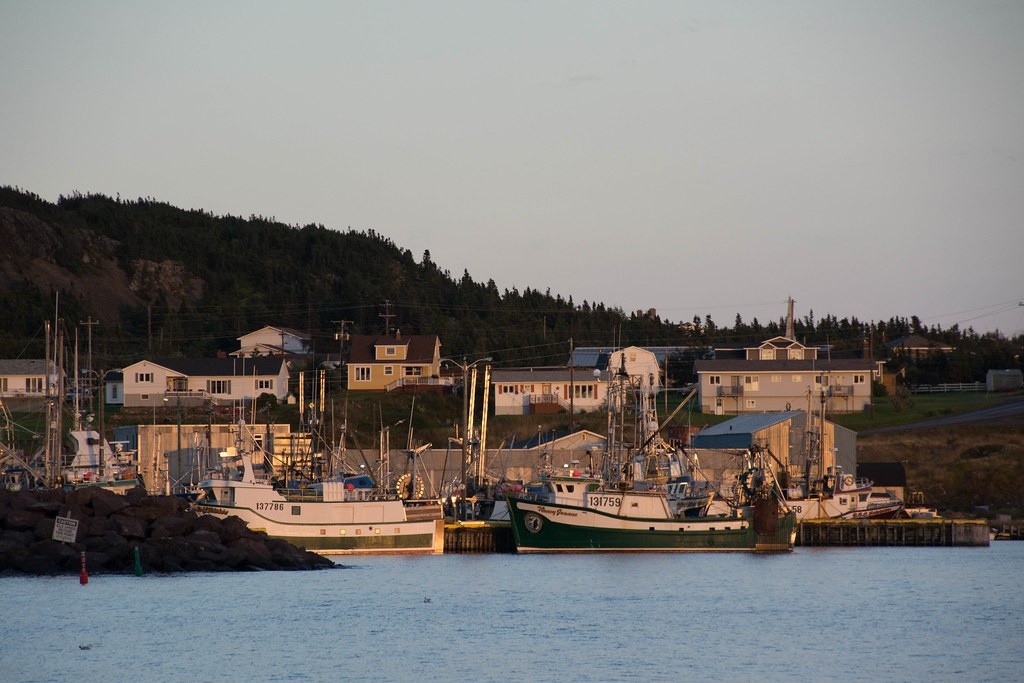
[0,288,943,553]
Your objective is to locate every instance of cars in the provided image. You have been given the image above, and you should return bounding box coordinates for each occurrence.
[65,389,94,401]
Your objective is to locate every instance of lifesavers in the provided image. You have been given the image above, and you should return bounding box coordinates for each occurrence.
[210,472,220,479]
[844,476,854,485]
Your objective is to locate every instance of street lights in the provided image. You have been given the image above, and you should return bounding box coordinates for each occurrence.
[436,358,493,521]
[80,369,124,480]
[163,388,182,491]
[592,369,614,487]
